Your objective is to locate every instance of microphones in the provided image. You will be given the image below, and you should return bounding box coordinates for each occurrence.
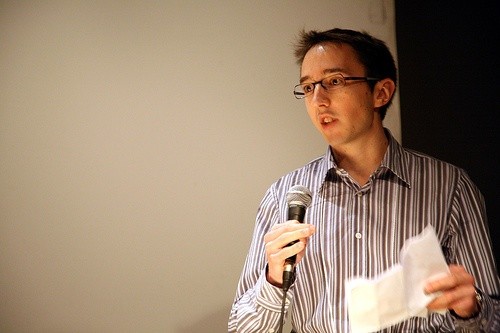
[283,184,314,290]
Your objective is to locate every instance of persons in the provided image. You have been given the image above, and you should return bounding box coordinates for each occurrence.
[227,25,500,333]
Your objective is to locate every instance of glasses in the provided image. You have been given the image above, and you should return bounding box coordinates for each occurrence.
[292,74,384,100]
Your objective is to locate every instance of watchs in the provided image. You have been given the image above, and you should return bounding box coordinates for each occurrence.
[474,288,482,313]
